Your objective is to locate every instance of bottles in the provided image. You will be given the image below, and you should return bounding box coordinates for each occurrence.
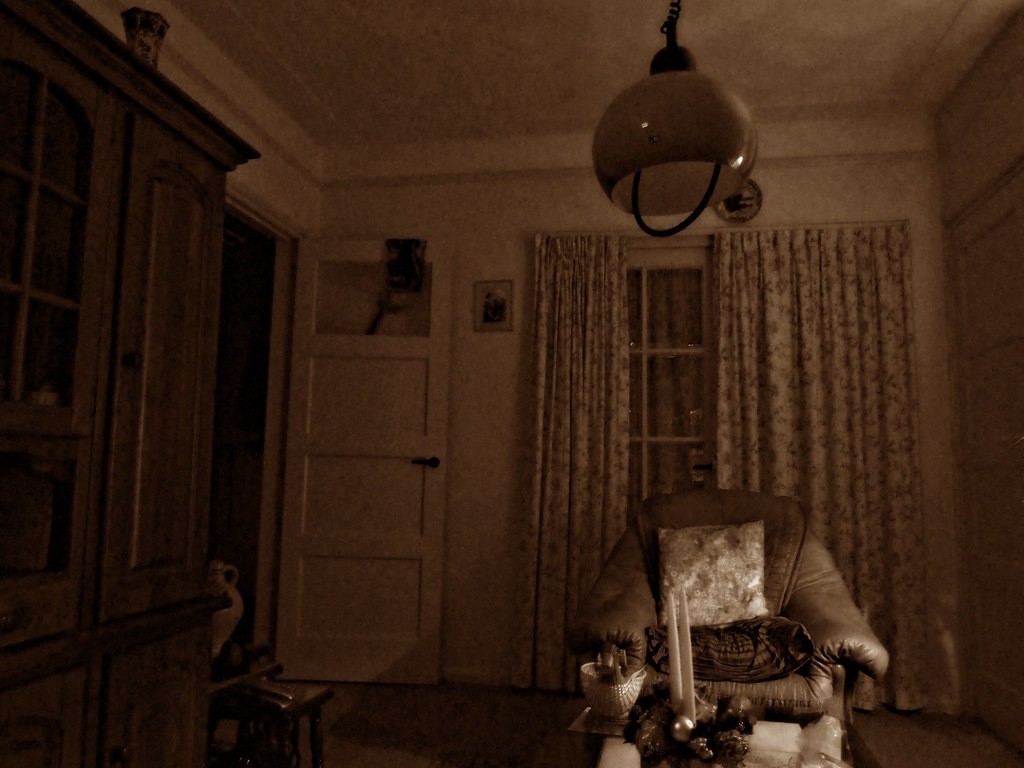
[210,561,243,658]
[685,445,705,490]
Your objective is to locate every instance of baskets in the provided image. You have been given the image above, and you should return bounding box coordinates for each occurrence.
[580,662,647,718]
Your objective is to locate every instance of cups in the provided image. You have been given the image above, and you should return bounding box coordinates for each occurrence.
[688,406,703,436]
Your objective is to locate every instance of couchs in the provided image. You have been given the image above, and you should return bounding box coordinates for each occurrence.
[561,489,893,739]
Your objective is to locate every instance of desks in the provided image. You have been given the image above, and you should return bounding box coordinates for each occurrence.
[204,679,336,768]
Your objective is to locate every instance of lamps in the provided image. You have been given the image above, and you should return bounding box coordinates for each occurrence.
[591,0,759,236]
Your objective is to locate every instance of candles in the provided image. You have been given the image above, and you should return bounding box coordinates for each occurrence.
[667,587,685,712]
[676,583,697,730]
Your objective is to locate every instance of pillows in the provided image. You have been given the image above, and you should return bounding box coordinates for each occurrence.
[655,519,771,633]
[644,614,816,683]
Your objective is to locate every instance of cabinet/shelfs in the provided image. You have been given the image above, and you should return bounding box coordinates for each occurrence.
[0,1,263,768]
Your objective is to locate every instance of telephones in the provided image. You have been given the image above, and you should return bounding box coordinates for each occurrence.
[234,677,306,713]
